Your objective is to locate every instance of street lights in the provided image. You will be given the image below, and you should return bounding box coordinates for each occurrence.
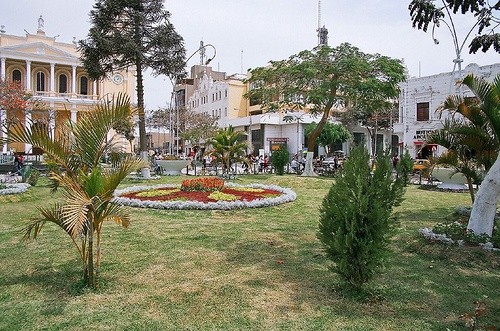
[170,40,217,159]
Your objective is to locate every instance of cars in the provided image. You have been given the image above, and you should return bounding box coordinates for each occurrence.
[412,158,430,172]
[315,155,347,167]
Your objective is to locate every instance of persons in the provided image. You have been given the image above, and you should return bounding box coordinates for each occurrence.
[11,152,19,176]
[392,154,399,170]
[152,149,340,175]
[18,153,24,170]
